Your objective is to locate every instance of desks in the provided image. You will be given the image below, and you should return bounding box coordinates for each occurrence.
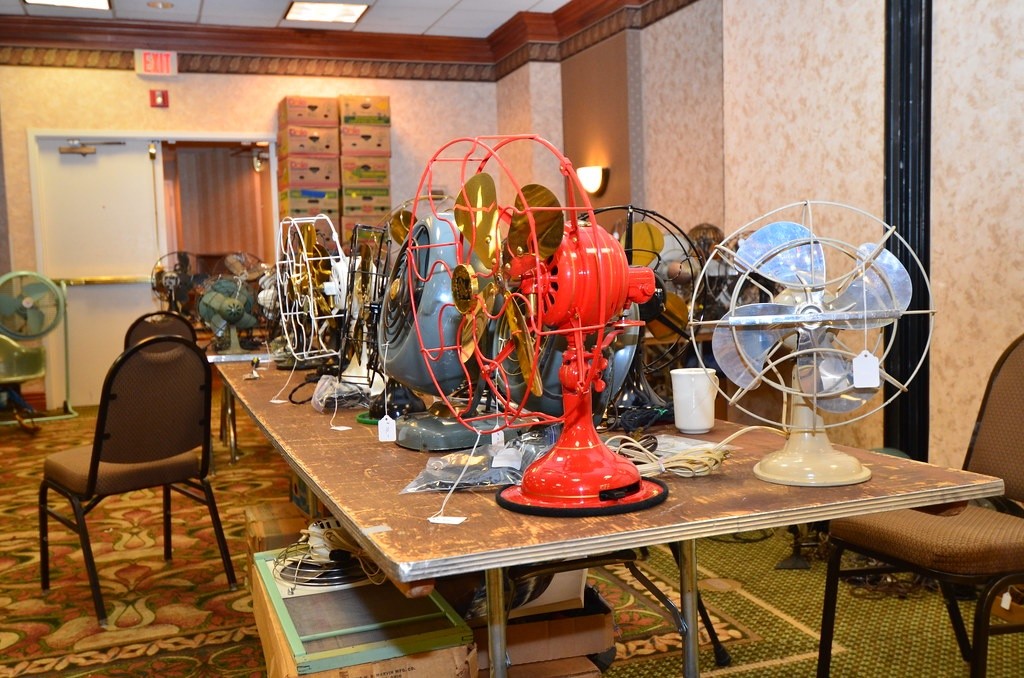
[197,338,1004,678]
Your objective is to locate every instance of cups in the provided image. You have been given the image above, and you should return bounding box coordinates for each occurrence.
[671,367,719,435]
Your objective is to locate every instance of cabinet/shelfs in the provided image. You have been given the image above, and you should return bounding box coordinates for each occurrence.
[643,333,798,431]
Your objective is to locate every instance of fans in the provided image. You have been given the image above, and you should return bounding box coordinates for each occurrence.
[406,134,668,518]
[549,206,704,422]
[152,196,641,452]
[273,516,385,596]
[0,271,79,425]
[688,200,937,489]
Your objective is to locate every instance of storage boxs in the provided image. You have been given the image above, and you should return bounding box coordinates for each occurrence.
[992,583,1024,624]
[277,95,392,256]
[245,469,614,677]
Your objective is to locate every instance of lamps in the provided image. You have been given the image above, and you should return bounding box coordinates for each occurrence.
[578,165,610,199]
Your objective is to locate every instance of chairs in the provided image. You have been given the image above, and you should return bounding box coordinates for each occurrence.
[124,312,197,349]
[0,335,47,384]
[817,335,1024,678]
[39,335,240,627]
